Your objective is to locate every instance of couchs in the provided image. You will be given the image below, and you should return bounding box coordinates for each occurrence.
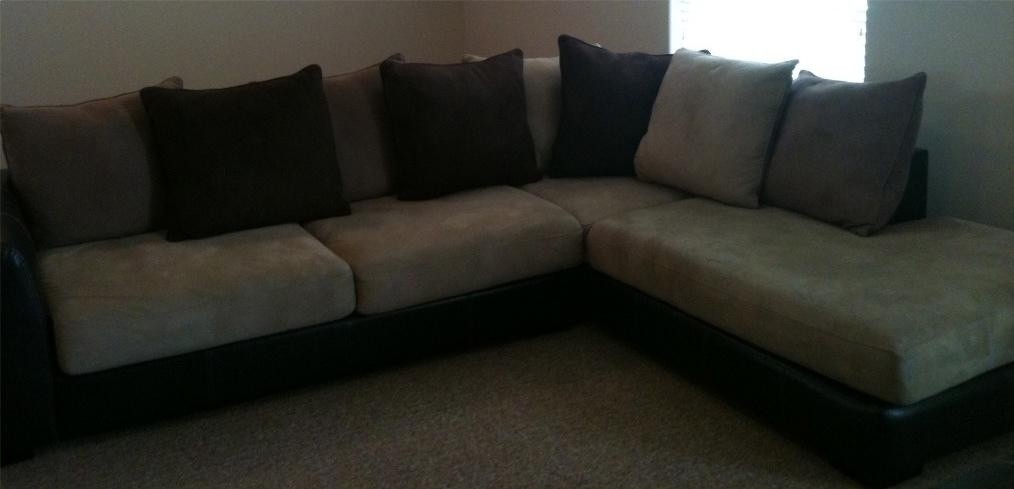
[1,147,1013,487]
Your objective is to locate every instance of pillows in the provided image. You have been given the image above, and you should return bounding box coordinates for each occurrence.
[378,47,535,202]
[548,34,710,177]
[321,51,406,204]
[1,76,184,251]
[139,64,352,242]
[632,47,800,210]
[460,44,602,181]
[760,69,927,236]
